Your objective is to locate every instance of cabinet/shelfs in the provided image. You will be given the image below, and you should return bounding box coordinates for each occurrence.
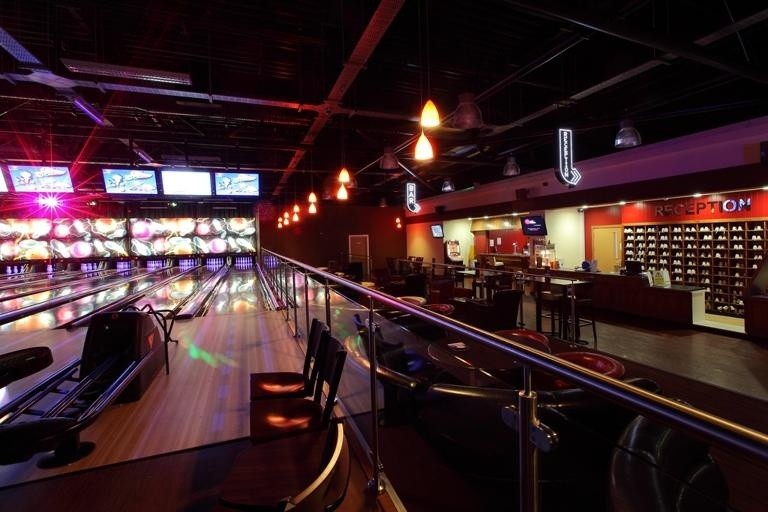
[623,220,768,316]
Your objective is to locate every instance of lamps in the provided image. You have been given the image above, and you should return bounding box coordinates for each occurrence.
[450,90,484,131]
[420,24,441,131]
[380,145,399,171]
[441,176,455,193]
[414,119,434,160]
[502,153,521,177]
[276,116,352,230]
[379,196,389,208]
[614,105,643,149]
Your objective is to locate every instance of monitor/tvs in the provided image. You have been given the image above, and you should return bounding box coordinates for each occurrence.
[0,164,12,192]
[7,164,76,194]
[101,166,159,199]
[520,216,547,236]
[213,169,262,198]
[159,168,216,200]
[431,224,444,238]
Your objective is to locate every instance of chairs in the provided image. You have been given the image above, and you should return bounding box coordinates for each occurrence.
[250,335,347,436]
[316,256,729,511]
[249,318,329,397]
